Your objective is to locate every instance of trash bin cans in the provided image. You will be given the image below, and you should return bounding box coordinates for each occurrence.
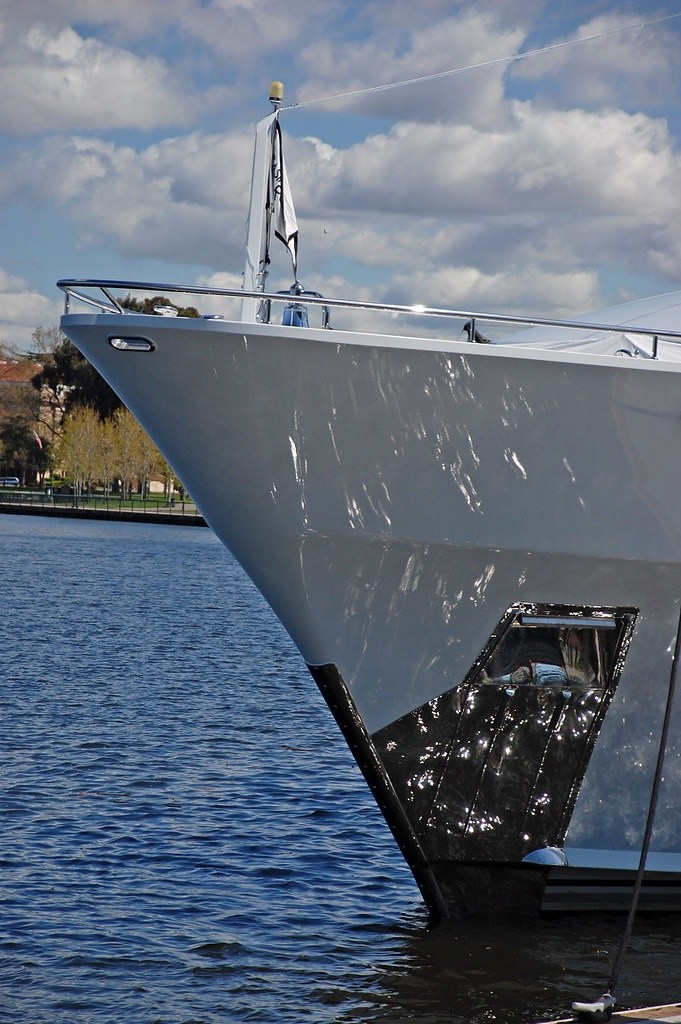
[46,487,60,499]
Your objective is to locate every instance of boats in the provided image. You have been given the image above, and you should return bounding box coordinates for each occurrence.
[55,78,681,931]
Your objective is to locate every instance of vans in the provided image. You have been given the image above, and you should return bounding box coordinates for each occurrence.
[0,476,20,486]
